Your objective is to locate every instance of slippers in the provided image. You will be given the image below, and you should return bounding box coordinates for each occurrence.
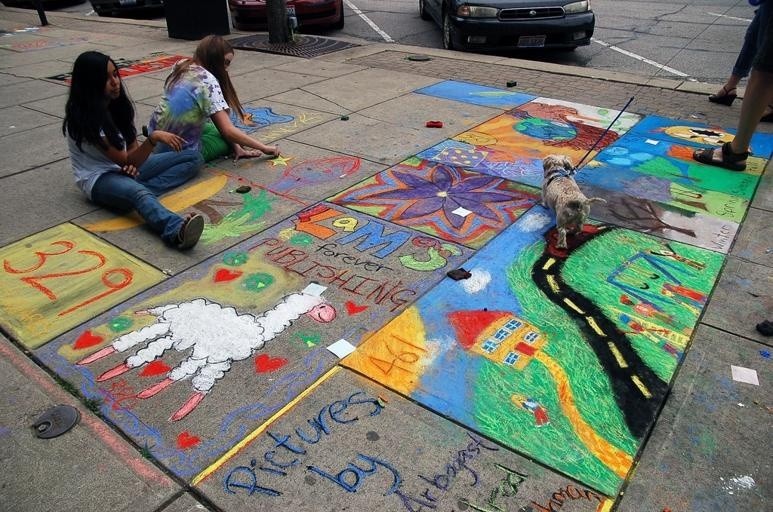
[178,211,205,252]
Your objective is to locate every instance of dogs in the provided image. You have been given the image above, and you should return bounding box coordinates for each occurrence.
[541,154,608,250]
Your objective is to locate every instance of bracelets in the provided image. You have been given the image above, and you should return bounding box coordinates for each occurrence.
[148,135,163,150]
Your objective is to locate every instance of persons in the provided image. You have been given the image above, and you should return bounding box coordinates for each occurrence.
[60,49,201,250]
[706,1,773,124]
[694,2,772,168]
[143,35,284,163]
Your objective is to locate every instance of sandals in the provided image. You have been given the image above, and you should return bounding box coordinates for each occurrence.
[693,141,749,172]
[709,85,737,107]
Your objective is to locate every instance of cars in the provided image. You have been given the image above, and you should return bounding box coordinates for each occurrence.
[417,0,595,54]
